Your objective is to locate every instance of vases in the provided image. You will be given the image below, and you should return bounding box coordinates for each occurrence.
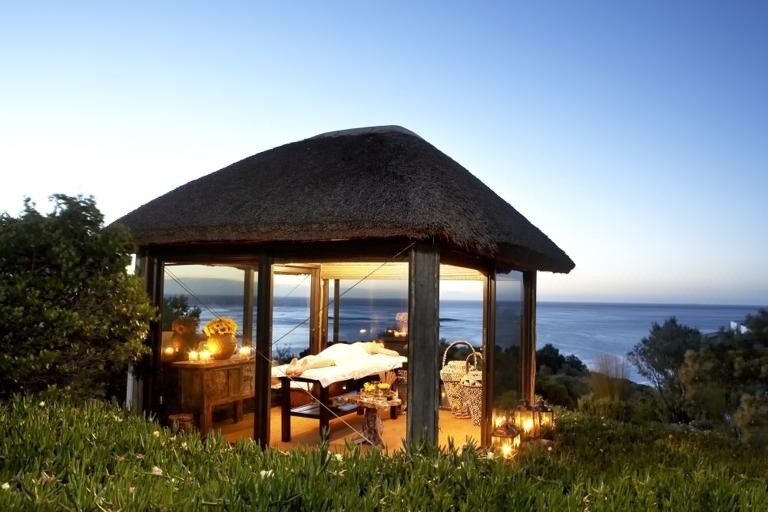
[206,334,238,360]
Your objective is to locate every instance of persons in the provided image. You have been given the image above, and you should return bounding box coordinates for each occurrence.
[286,337,399,382]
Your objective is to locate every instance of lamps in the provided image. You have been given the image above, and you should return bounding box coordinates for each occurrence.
[491,399,553,459]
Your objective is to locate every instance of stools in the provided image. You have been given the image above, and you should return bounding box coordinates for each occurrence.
[168,412,194,435]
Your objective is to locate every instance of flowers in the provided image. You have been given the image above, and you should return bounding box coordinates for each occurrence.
[204,318,237,336]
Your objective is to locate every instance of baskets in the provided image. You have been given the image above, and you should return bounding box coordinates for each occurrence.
[461,351,483,427]
[440,340,477,420]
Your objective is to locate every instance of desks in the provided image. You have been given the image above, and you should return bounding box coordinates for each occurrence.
[171,359,256,436]
[342,389,402,449]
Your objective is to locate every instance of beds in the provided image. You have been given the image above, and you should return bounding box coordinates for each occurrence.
[271,342,409,448]
